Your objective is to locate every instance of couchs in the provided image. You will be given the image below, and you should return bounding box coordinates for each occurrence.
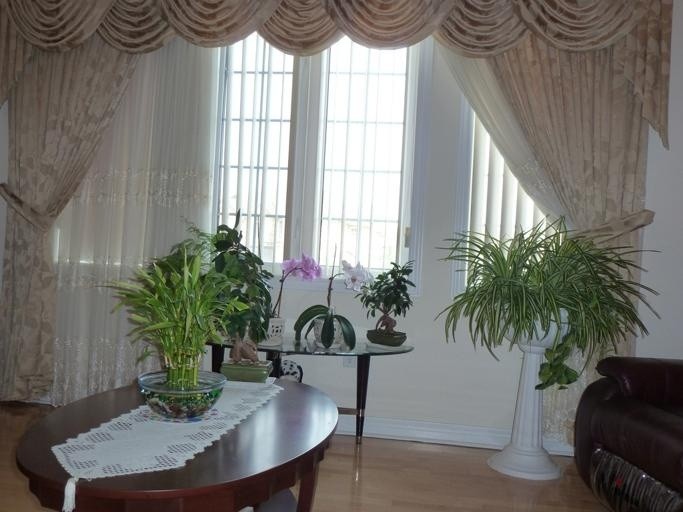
[574,357,683,512]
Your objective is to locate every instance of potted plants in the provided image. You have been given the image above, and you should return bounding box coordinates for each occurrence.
[87,244,251,418]
[210,211,274,383]
[433,215,663,484]
[354,260,417,346]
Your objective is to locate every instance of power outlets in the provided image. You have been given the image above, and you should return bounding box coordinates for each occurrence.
[342,356,356,368]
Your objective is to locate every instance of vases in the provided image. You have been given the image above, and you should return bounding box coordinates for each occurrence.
[257,316,284,348]
[314,320,344,350]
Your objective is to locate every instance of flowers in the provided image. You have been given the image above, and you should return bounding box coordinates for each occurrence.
[254,253,323,347]
[293,246,375,350]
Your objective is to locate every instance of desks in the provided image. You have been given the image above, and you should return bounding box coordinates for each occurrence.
[204,326,414,445]
[13,378,339,511]
